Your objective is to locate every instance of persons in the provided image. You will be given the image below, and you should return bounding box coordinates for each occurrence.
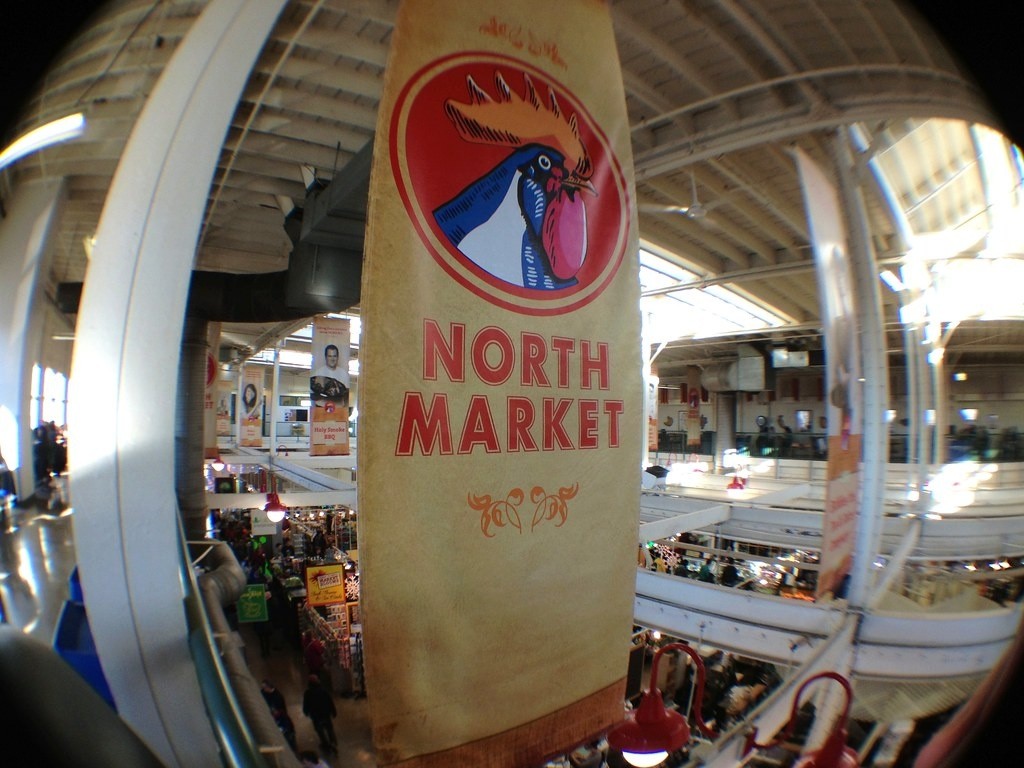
[647,413,1022,464]
[545,735,612,768]
[310,345,352,399]
[635,532,821,594]
[32,425,68,508]
[682,649,779,734]
[209,506,365,768]
[240,384,261,419]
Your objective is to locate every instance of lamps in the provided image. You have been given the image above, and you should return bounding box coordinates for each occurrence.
[201,445,358,538]
[0,104,88,171]
[609,642,720,768]
[736,672,864,768]
